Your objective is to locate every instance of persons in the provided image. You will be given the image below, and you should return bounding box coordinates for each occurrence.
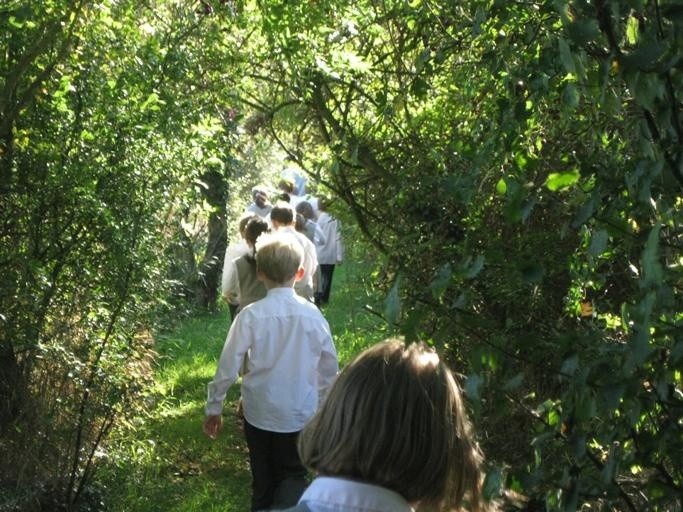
[219,153,343,322]
[276,335,485,511]
[199,239,339,512]
